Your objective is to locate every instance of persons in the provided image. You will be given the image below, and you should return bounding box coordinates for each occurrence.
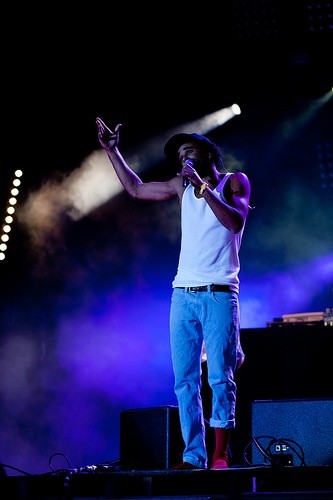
[96,116,251,470]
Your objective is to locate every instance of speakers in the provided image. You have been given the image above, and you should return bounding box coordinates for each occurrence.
[250,398,333,467]
[120,405,232,471]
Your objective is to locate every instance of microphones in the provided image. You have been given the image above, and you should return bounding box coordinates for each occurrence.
[182,159,194,187]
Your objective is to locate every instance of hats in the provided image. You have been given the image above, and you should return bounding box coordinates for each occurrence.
[164,133,220,162]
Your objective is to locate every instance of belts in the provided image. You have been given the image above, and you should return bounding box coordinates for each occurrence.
[176,284,233,292]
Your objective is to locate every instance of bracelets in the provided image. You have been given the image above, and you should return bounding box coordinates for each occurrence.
[199,182,207,196]
